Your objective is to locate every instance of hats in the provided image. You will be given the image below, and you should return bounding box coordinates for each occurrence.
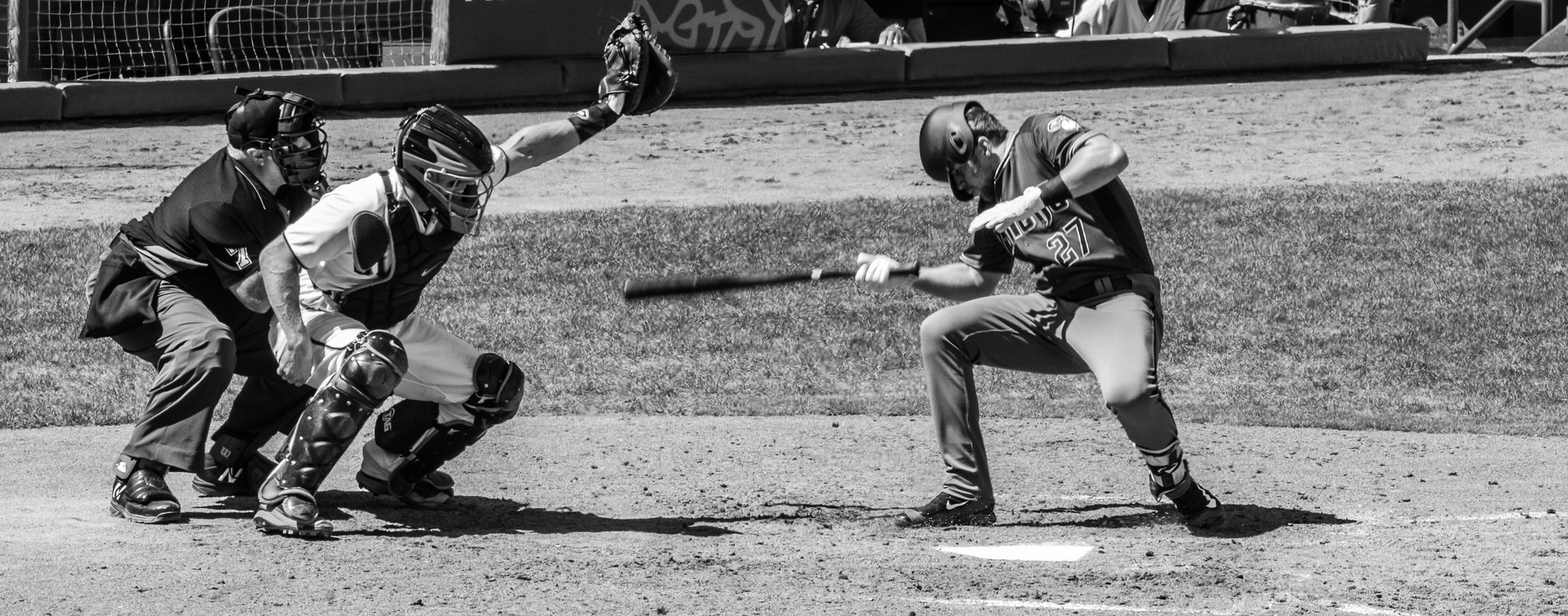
[228,95,283,143]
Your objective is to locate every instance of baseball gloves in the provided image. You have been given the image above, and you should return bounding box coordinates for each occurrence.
[598,13,679,116]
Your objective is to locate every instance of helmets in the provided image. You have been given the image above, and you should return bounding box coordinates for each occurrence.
[396,100,494,239]
[920,101,985,201]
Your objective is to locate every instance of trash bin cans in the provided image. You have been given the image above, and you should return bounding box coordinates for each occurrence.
[1226,0,1331,31]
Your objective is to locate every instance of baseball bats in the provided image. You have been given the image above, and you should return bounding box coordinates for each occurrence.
[623,259,920,301]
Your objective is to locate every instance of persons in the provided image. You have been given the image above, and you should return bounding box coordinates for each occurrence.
[257,14,677,539]
[855,102,1225,531]
[79,86,329,525]
[782,0,1240,48]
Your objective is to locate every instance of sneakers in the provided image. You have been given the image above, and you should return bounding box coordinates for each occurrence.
[894,491,997,529]
[192,451,278,496]
[1172,476,1224,527]
[110,467,181,523]
[253,495,333,537]
[356,469,459,511]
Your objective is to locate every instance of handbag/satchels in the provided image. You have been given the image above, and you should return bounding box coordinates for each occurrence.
[79,236,162,338]
